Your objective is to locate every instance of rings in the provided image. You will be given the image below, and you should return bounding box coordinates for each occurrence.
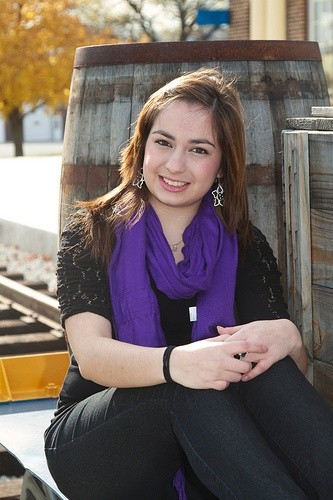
[233,352,246,360]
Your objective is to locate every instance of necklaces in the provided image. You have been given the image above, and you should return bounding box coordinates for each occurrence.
[167,239,183,254]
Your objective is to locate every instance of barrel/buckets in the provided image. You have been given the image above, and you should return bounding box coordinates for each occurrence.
[60,40,330,252]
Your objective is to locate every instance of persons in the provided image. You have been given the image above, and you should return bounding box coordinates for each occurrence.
[42,66,333,500]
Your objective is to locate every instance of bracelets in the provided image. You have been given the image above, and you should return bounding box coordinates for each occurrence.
[163,346,177,386]
